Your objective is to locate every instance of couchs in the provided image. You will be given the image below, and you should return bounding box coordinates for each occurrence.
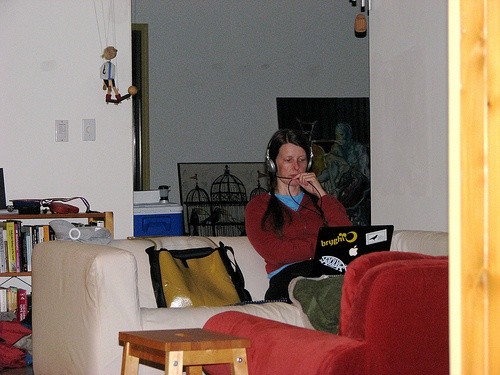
[32,230,450,375]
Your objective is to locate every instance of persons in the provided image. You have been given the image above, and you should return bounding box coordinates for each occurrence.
[245,127,354,304]
[100,47,122,101]
[307,122,369,199]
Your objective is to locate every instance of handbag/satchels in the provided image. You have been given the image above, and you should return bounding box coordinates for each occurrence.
[144,241,252,309]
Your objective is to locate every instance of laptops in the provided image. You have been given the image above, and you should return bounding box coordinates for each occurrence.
[310,225,394,278]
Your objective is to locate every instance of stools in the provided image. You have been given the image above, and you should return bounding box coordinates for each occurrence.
[119,328,251,375]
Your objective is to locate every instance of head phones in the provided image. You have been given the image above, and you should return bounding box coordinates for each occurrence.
[265,129,314,174]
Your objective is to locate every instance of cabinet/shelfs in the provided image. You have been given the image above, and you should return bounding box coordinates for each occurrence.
[0,212,114,325]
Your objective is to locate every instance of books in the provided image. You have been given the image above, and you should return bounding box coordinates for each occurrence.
[0,286,28,322]
[0,220,105,273]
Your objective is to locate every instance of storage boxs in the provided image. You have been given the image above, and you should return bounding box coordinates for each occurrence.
[134,203,183,236]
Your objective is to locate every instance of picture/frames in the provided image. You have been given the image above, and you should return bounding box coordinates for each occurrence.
[177,161,271,236]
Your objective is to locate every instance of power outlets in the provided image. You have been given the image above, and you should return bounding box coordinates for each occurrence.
[55,120,68,143]
